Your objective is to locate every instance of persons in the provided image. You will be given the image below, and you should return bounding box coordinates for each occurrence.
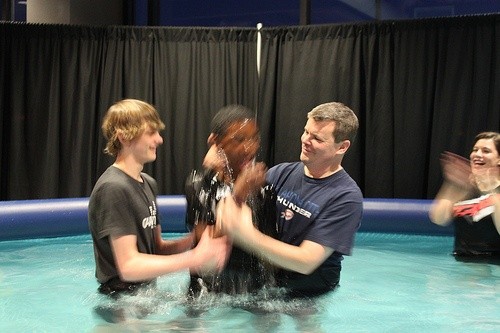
[202,102,364,298]
[184,104,276,297]
[428,132,500,255]
[89,98,229,311]
